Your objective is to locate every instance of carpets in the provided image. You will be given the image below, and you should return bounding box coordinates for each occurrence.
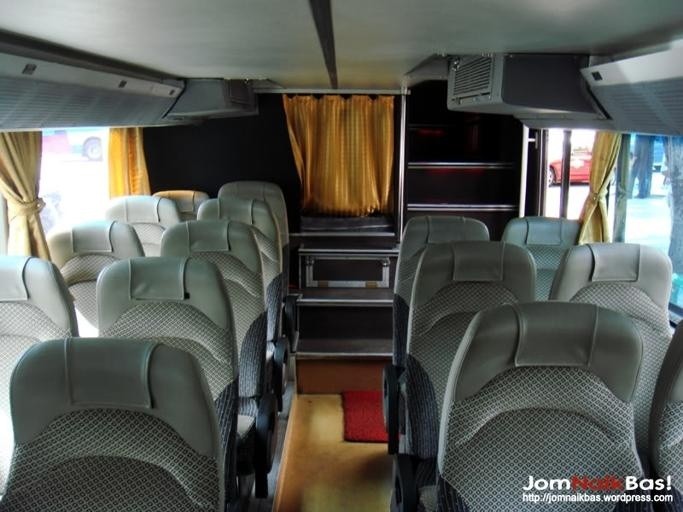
[340,388,401,443]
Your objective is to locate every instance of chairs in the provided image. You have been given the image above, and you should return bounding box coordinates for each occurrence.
[383,213,683,512]
[0,182,300,512]
